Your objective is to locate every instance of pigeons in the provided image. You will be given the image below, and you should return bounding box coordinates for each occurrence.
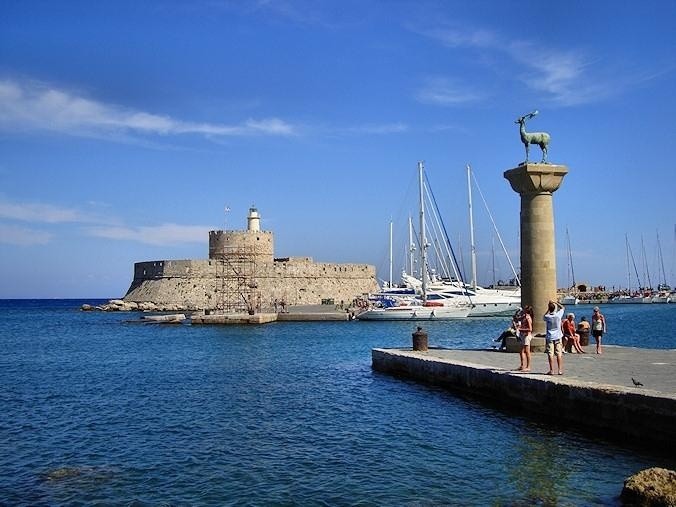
[631,376,644,387]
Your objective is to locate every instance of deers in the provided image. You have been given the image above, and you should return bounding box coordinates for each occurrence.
[514,110,550,164]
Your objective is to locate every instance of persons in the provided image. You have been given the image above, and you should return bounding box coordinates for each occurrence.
[336,296,382,313]
[590,306,606,354]
[559,314,568,354]
[272,298,289,314]
[492,309,523,350]
[562,313,585,354]
[515,305,534,372]
[576,316,590,332]
[543,300,564,376]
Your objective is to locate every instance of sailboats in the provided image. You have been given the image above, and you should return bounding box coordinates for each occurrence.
[610,229,676,303]
[359,163,524,320]
[559,224,581,305]
